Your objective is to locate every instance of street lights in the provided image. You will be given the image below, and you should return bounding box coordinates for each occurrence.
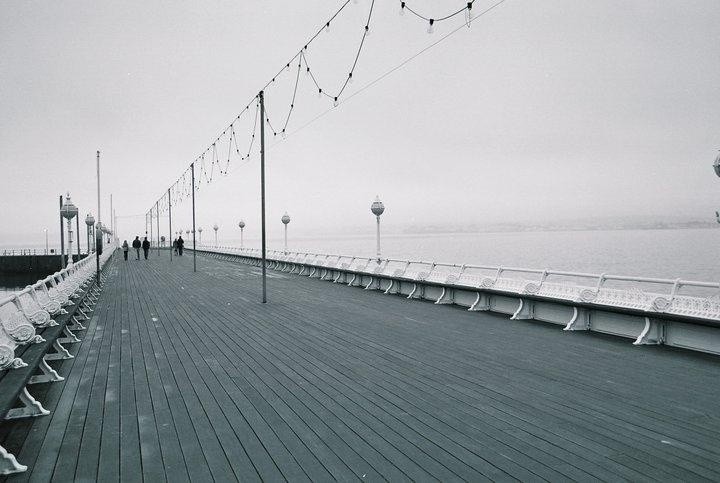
[214,225,218,241]
[281,211,291,249]
[85,212,95,255]
[96,222,111,248]
[239,219,245,247]
[175,227,202,242]
[62,193,78,266]
[371,196,385,258]
[44,227,49,255]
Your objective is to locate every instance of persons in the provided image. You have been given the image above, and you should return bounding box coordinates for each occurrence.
[123,236,150,261]
[173,236,184,256]
